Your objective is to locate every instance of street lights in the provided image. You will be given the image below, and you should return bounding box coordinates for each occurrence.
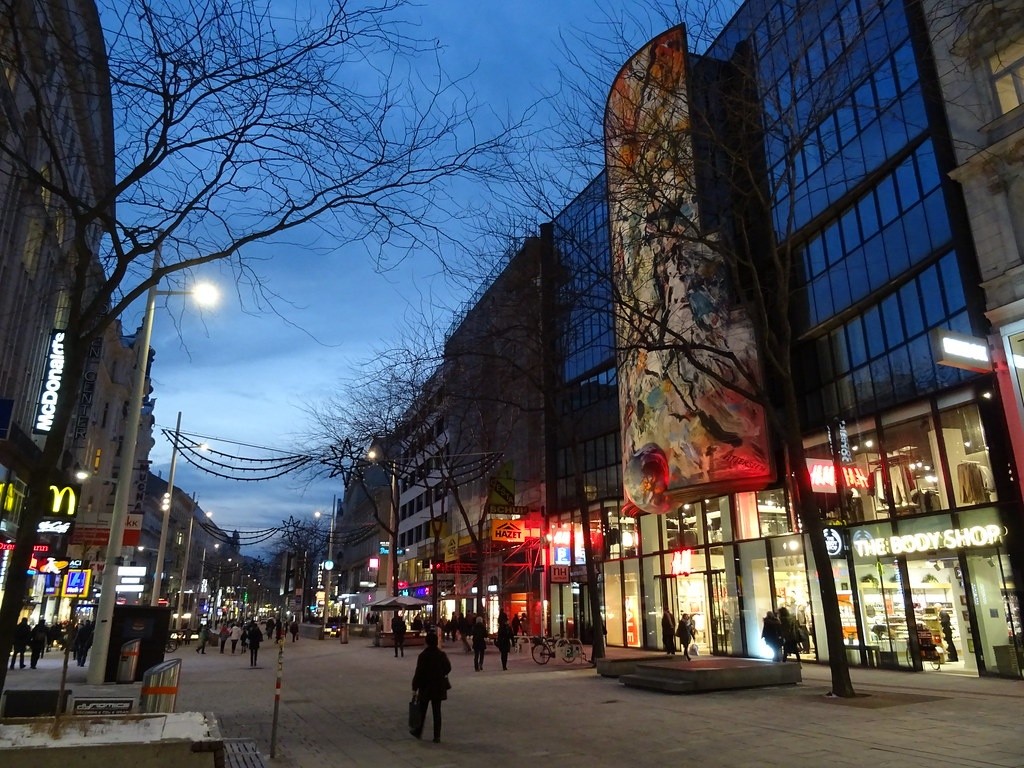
[188,544,220,630]
[236,574,250,622]
[208,558,232,630]
[170,511,213,630]
[138,444,208,606]
[315,511,341,624]
[369,451,409,632]
[77,288,218,686]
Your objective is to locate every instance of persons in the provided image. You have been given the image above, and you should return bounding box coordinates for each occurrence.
[391,616,406,657]
[195,625,209,654]
[219,623,229,653]
[240,631,248,654]
[410,633,451,743]
[662,607,803,668]
[266,620,273,639]
[9,617,31,670]
[46,624,56,651]
[366,612,379,624]
[512,613,528,635]
[498,609,515,671]
[274,619,282,636]
[588,619,607,663]
[411,610,488,671]
[248,624,264,667]
[274,625,280,644]
[231,621,236,628]
[229,623,242,655]
[74,622,93,667]
[290,622,299,642]
[76,620,84,628]
[71,625,83,660]
[28,620,51,669]
[939,610,959,661]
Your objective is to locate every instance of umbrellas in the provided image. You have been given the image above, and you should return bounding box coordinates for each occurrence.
[371,595,430,621]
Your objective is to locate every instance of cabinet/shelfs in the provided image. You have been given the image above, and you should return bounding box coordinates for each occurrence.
[858,584,954,651]
[863,448,921,518]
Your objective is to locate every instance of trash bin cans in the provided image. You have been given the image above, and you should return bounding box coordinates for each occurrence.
[117,638,142,684]
[431,624,442,650]
[139,658,181,713]
[340,622,350,644]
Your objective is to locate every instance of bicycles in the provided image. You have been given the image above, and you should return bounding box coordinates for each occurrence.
[531,631,576,665]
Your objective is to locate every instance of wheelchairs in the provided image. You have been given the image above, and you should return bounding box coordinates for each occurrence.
[905,629,941,670]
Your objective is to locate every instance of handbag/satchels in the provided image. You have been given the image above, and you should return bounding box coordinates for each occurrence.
[445,677,451,691]
[409,695,422,729]
[689,640,699,656]
